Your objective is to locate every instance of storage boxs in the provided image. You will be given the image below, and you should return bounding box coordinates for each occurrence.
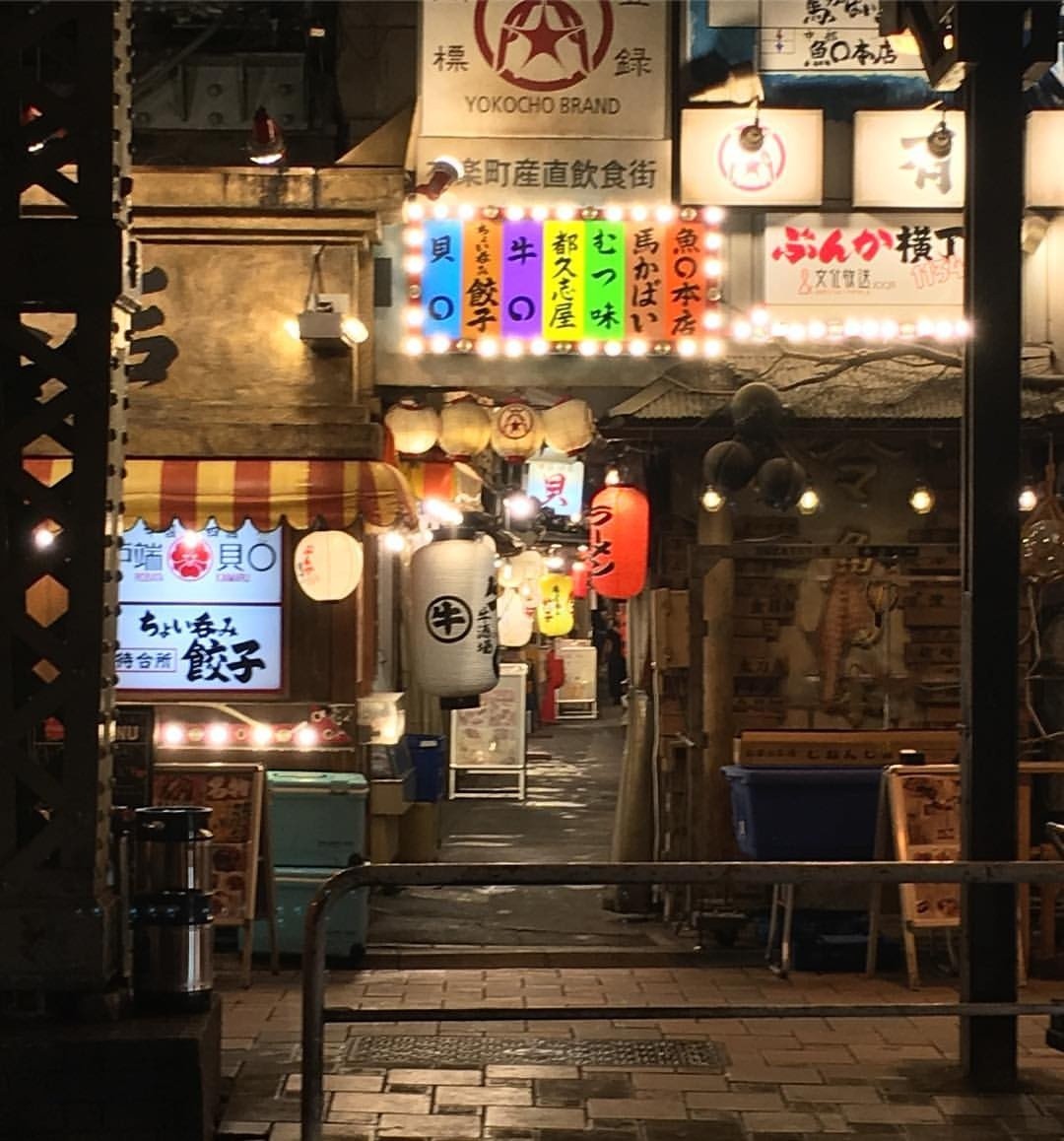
[234,769,375,969]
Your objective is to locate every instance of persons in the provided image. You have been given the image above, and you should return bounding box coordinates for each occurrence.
[593,600,628,706]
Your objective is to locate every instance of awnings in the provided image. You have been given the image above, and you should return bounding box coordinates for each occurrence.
[120,458,420,534]
[401,461,460,503]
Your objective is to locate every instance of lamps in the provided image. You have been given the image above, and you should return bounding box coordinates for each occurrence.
[243,100,294,168]
[400,150,464,202]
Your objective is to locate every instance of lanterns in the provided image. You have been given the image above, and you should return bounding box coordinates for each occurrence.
[490,402,544,463]
[383,399,442,454]
[436,399,492,462]
[409,542,499,710]
[527,460,584,523]
[496,549,589,648]
[544,395,597,458]
[588,484,647,599]
[293,532,365,602]
